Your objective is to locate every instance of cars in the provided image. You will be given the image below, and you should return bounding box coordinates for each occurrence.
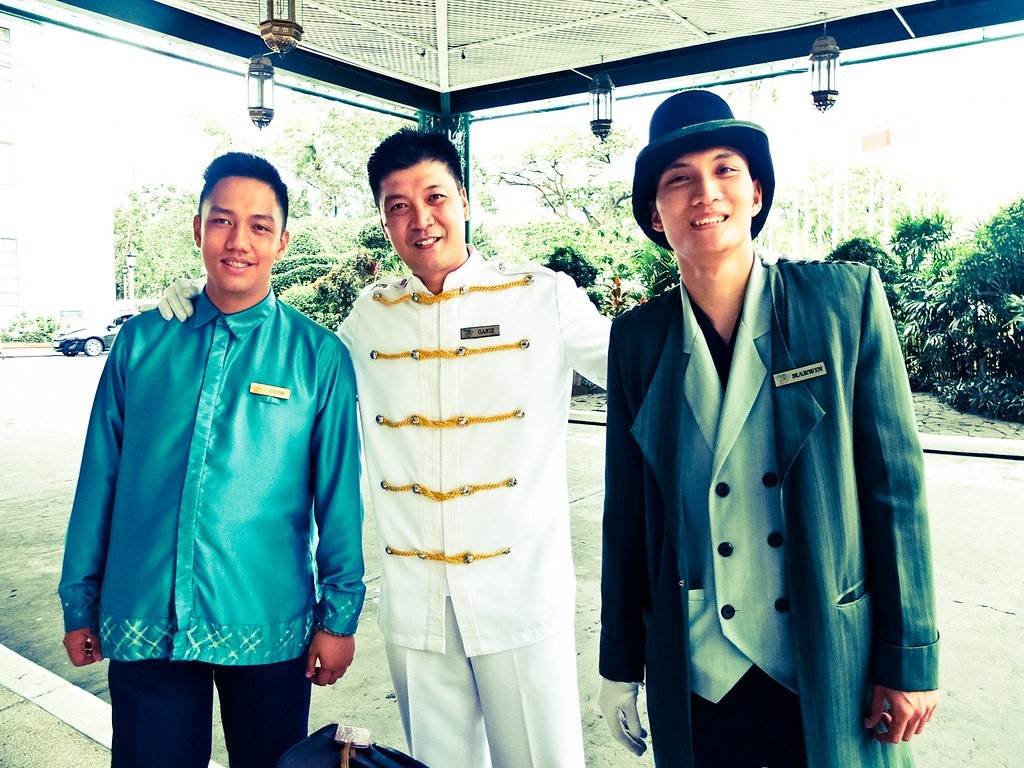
[52,300,161,357]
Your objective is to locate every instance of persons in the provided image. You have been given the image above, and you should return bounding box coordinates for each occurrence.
[57,152,365,768]
[597,89,940,768]
[156,129,788,768]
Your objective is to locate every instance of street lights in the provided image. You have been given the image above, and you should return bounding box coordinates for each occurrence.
[122,266,128,299]
[126,252,137,299]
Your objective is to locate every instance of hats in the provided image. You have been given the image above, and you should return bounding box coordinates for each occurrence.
[631,90,775,250]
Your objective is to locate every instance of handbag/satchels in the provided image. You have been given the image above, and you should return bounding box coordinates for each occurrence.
[275,724,428,768]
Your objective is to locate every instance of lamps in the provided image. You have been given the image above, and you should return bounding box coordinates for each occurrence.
[258,0,304,58]
[587,57,613,141]
[247,36,275,131]
[807,12,840,111]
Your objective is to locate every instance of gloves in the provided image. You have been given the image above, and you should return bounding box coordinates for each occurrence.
[139,278,204,323]
[597,677,647,757]
